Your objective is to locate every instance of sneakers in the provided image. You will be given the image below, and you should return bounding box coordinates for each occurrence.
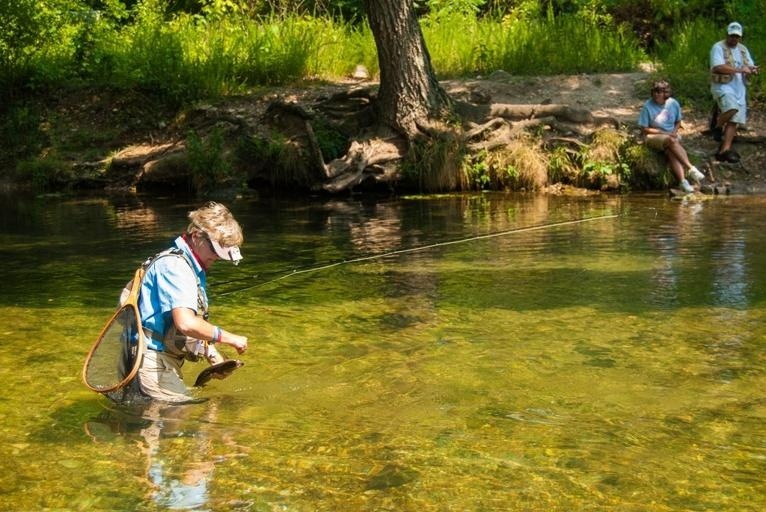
[717,149,740,163]
[679,180,694,193]
[686,165,705,181]
[710,124,722,141]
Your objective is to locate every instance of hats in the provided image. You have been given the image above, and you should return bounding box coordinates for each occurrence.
[728,22,743,37]
[193,218,244,266]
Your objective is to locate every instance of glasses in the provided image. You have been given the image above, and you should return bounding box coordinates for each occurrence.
[652,90,665,94]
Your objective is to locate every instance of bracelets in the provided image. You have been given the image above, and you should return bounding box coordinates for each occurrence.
[211,325,218,345]
[216,326,224,343]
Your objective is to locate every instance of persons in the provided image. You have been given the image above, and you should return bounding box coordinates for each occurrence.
[708,210,752,314]
[637,78,705,195]
[705,20,761,163]
[140,400,240,512]
[113,200,246,441]
[645,199,707,308]
